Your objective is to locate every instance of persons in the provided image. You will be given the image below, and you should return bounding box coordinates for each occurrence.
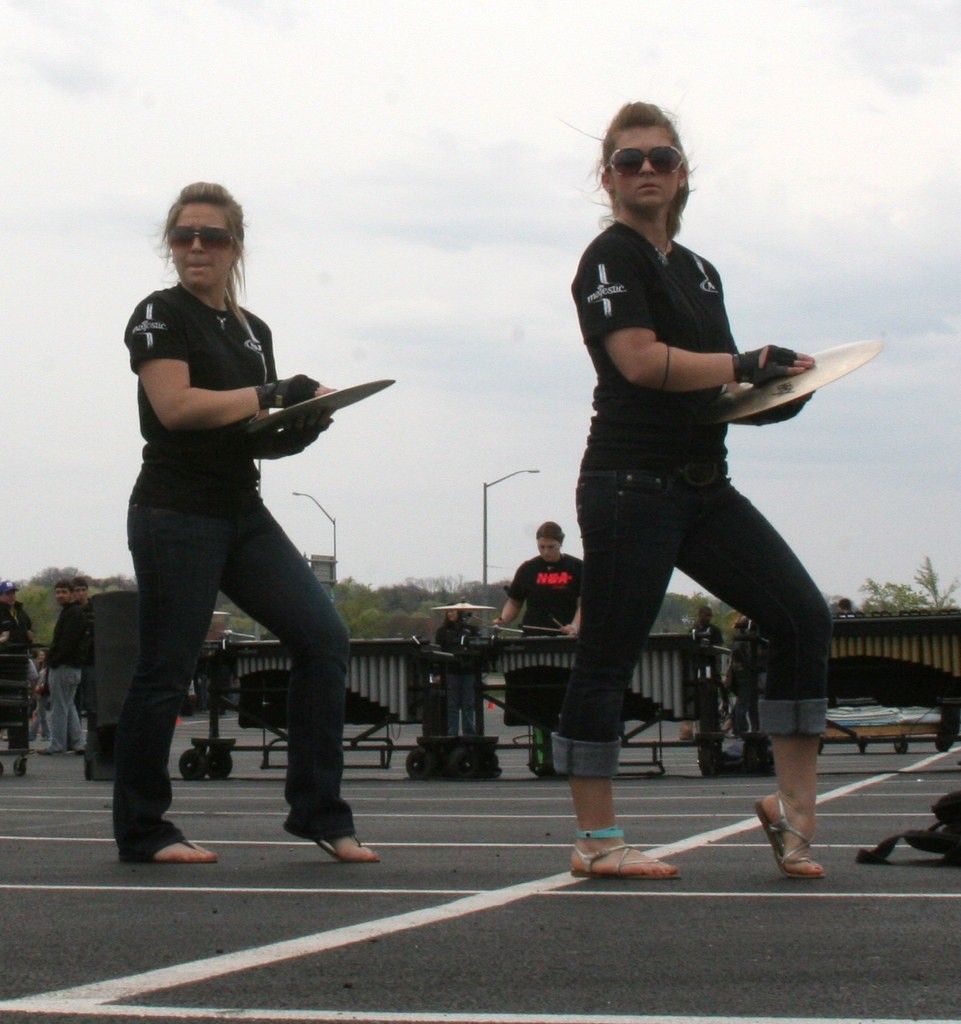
[491,521,584,778]
[551,100,832,878]
[28,649,50,741]
[837,599,852,613]
[69,577,95,713]
[0,580,34,754]
[433,603,483,736]
[721,615,767,739]
[112,183,382,862]
[37,580,88,755]
[679,606,725,740]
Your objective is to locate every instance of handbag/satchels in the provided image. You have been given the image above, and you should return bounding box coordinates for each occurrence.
[36,686,49,696]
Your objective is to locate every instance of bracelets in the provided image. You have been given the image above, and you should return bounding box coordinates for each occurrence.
[570,622,580,633]
[656,345,670,391]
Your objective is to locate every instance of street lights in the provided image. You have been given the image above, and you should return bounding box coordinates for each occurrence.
[482,470,540,637]
[292,492,337,606]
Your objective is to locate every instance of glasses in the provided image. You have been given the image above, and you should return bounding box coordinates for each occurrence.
[171,225,230,247]
[605,146,686,176]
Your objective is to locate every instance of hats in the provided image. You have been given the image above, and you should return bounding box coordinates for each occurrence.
[53,579,73,591]
[0,581,19,593]
[73,577,89,589]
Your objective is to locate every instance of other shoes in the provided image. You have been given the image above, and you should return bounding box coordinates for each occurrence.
[37,748,63,756]
[76,747,85,755]
[679,725,692,740]
[37,735,49,742]
[2,734,8,741]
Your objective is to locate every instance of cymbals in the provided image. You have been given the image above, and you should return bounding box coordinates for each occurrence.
[244,376,398,434]
[695,337,885,429]
[429,602,496,612]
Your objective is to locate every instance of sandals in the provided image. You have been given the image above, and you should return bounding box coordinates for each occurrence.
[120,828,219,865]
[569,829,681,879]
[285,813,381,863]
[753,794,827,879]
